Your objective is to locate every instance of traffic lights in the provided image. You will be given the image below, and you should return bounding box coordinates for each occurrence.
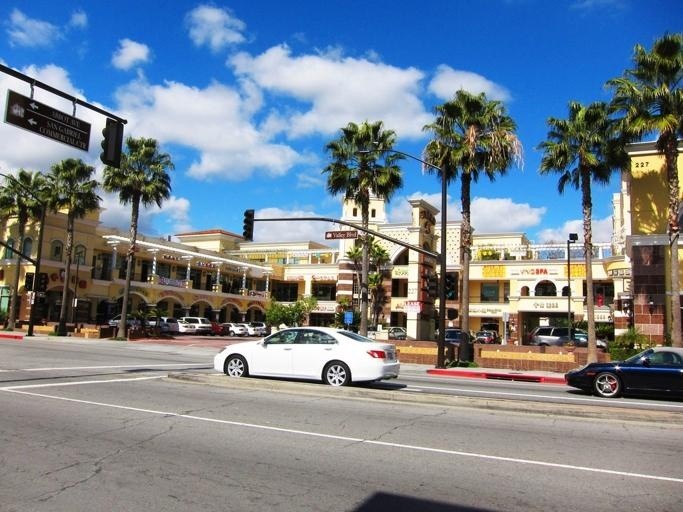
[100,118,107,166]
[242,209,254,241]
[36,293,46,304]
[427,274,436,298]
[442,271,456,299]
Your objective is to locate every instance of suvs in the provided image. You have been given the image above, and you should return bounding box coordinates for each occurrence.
[525,326,605,349]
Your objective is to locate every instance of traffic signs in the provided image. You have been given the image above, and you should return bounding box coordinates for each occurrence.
[0,89,90,154]
[324,230,358,238]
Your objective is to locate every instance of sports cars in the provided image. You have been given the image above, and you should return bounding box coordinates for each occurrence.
[564,346,682,398]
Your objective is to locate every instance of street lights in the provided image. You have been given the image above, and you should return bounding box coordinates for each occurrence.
[567,233,577,348]
[71,248,87,329]
[350,147,446,370]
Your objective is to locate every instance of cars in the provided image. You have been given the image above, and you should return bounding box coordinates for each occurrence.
[213,326,401,386]
[434,326,502,345]
[338,325,407,341]
[105,313,294,337]
